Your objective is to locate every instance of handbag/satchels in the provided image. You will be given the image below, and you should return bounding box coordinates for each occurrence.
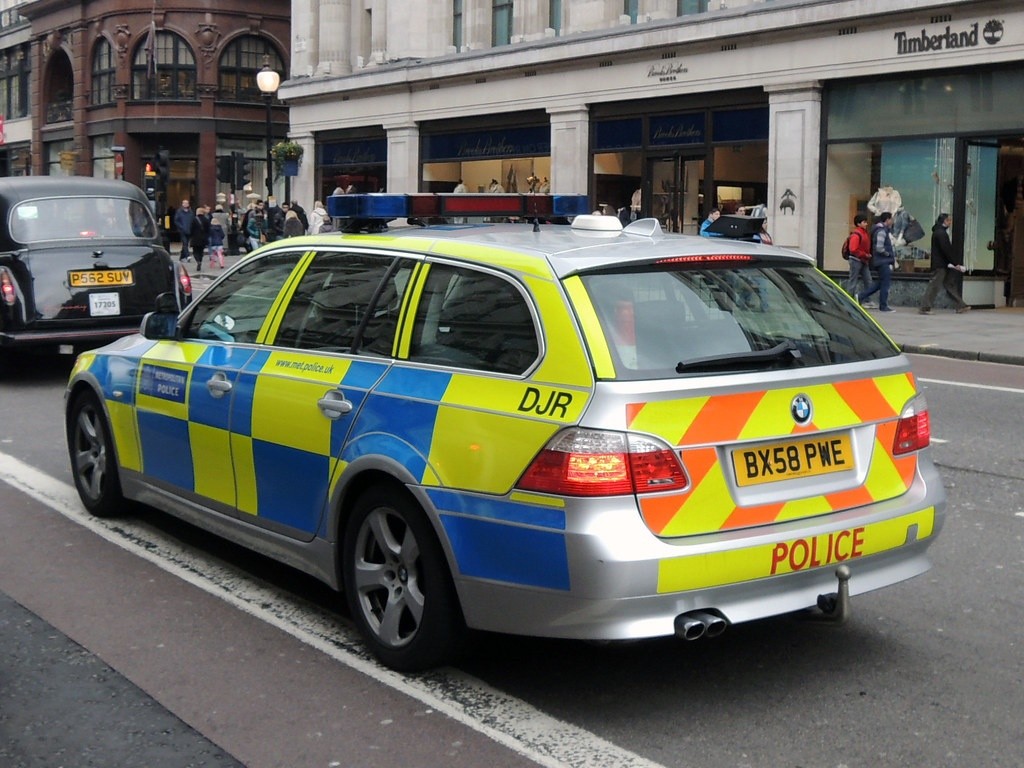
[903,216,924,243]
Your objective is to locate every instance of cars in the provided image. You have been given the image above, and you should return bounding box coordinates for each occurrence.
[0,176,193,350]
[62,193,949,680]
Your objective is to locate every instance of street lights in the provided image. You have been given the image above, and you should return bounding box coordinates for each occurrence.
[110,147,125,180]
[256,62,280,242]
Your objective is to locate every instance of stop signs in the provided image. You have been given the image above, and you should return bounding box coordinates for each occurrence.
[115,154,123,174]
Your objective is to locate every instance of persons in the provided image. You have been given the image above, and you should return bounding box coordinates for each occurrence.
[239,199,268,253]
[527,171,550,193]
[855,212,896,313]
[174,199,194,262]
[700,203,773,313]
[918,213,972,315]
[210,218,225,270]
[630,186,642,220]
[848,214,879,309]
[273,200,309,239]
[309,200,334,235]
[211,204,229,255]
[453,178,468,224]
[194,204,214,271]
[867,185,911,270]
[483,178,505,222]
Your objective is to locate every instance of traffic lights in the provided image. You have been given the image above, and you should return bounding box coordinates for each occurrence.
[231,151,251,190]
[217,156,230,183]
[154,152,169,181]
[144,161,155,178]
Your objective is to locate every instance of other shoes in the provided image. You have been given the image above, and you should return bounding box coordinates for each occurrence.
[856,293,861,306]
[880,306,896,313]
[187,256,191,262]
[864,301,879,309]
[197,262,201,271]
[956,305,971,313]
[920,307,936,315]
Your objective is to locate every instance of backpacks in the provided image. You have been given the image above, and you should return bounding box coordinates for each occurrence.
[842,232,862,260]
